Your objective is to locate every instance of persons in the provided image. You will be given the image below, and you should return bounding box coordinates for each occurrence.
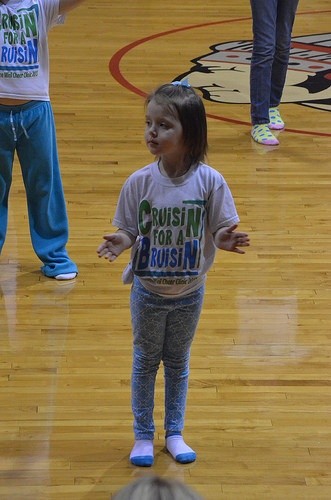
[250,0,299,145]
[0,0,81,281]
[95,83,250,467]
[108,474,205,500]
